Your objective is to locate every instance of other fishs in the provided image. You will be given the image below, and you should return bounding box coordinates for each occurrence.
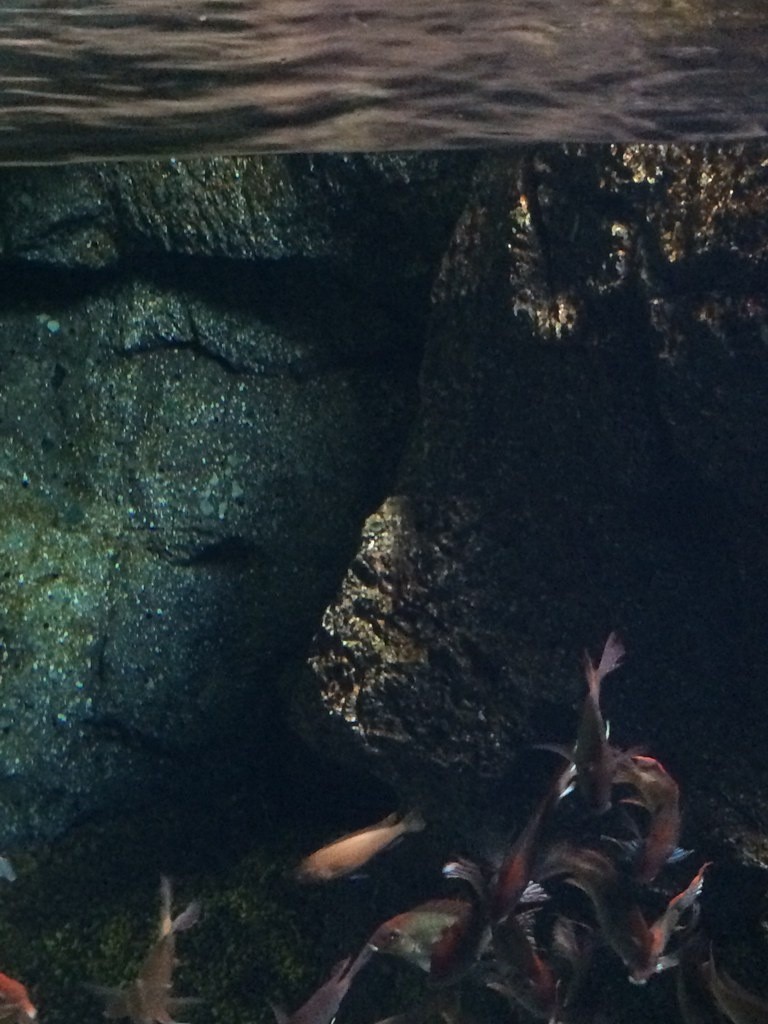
[0,630,768,1024]
